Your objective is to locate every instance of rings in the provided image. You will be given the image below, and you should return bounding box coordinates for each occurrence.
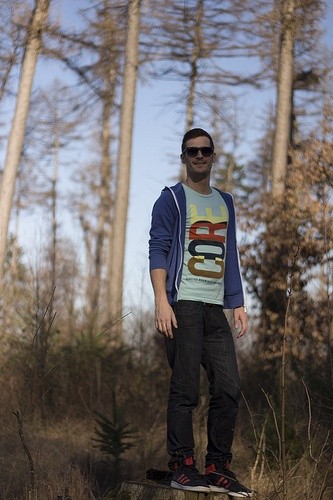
[161,323,167,327]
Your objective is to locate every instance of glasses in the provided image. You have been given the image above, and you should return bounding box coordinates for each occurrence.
[183,147,215,158]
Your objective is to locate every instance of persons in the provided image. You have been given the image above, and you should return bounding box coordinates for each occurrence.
[149,128,261,498]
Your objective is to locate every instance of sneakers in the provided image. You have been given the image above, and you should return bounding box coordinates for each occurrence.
[203,460,257,497]
[169,455,211,491]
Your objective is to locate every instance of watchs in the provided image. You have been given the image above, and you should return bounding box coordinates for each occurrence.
[234,305,247,313]
